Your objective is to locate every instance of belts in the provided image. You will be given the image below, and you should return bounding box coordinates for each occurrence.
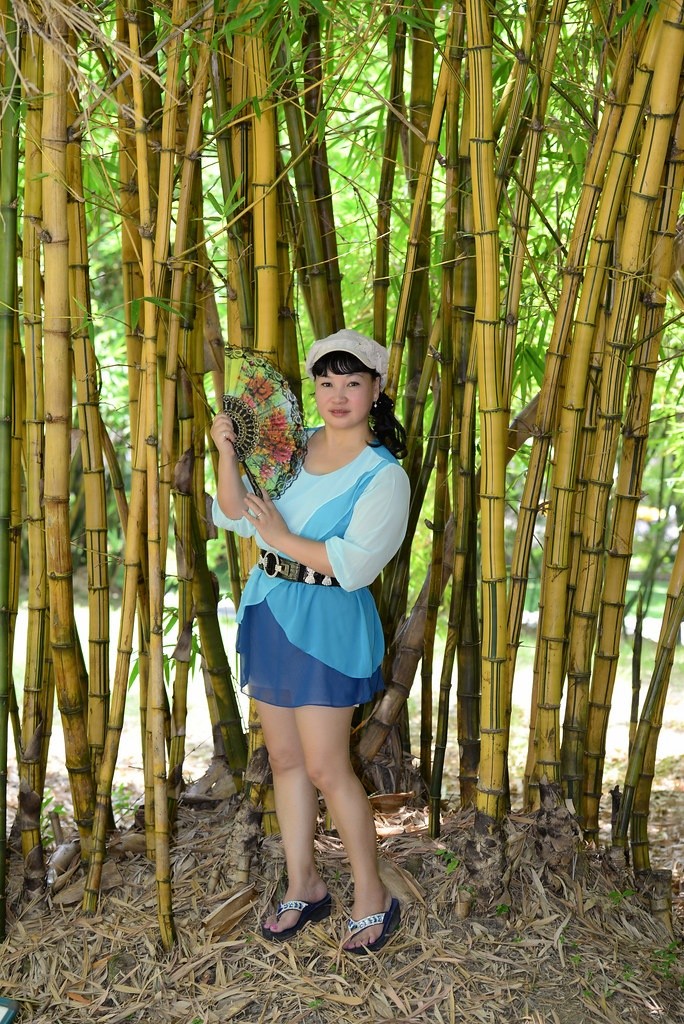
[258,549,341,587]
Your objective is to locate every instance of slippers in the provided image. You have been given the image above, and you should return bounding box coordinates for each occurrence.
[261,890,332,942]
[341,897,401,956]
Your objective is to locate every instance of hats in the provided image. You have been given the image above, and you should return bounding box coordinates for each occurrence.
[304,328,390,393]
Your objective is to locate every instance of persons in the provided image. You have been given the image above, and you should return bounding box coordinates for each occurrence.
[210,328,412,957]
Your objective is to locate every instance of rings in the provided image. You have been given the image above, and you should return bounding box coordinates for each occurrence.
[223,431,226,438]
[257,511,264,517]
